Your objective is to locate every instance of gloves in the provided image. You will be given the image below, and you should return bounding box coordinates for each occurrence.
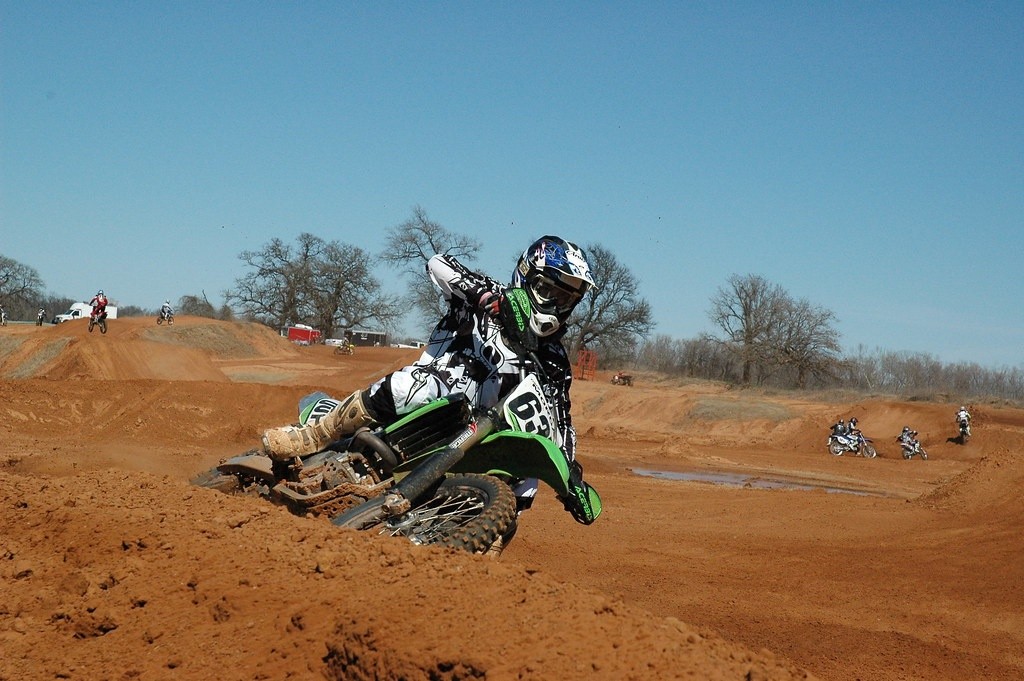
[477,292,510,327]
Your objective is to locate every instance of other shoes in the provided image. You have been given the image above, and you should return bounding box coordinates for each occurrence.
[915,449,919,453]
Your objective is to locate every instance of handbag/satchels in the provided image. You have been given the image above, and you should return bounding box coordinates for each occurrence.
[344,340,349,347]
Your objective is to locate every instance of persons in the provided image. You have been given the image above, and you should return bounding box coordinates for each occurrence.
[898,426,921,453]
[826,417,861,451]
[0,304,4,322]
[89,289,108,320]
[956,406,972,436]
[160,299,172,320]
[261,236,599,559]
[36,307,46,325]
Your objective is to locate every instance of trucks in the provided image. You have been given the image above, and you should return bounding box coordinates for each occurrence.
[51,303,118,325]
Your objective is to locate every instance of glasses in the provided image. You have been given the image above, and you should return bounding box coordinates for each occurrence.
[526,271,581,316]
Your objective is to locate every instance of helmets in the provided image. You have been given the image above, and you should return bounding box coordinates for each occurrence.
[903,426,909,431]
[166,300,170,303]
[960,407,965,410]
[850,417,858,423]
[512,235,599,337]
[97,290,103,294]
[839,419,844,423]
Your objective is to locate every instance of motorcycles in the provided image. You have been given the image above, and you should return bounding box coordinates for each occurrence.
[955,411,974,444]
[0,307,8,326]
[156,309,174,325]
[189,281,603,554]
[609,371,636,387]
[35,313,46,326]
[87,304,108,334]
[899,434,928,461]
[333,339,355,356]
[826,430,877,460]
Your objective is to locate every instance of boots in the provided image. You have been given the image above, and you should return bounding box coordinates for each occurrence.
[261,390,386,458]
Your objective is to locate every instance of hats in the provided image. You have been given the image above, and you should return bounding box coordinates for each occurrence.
[344,334,349,337]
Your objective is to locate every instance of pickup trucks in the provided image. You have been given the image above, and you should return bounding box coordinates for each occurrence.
[397,341,426,349]
